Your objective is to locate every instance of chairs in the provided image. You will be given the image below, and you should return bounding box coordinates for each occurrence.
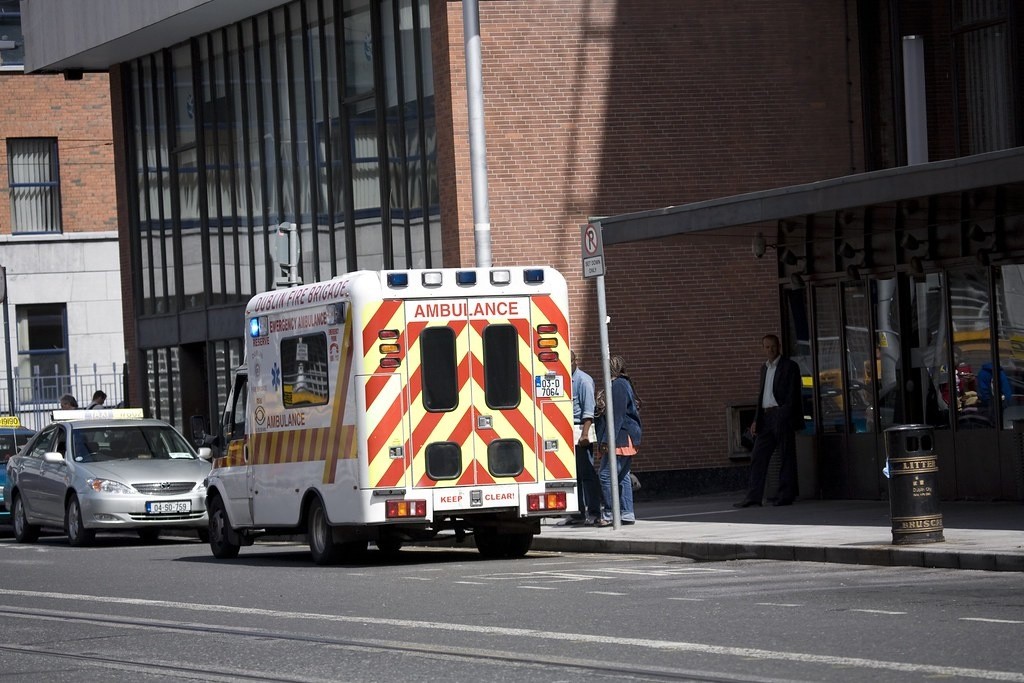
[122,432,149,458]
[86,441,106,463]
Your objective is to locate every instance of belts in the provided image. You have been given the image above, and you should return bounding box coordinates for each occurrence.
[763,406,781,412]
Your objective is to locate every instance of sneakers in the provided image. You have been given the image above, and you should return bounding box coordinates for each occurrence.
[584,514,598,526]
[557,515,586,526]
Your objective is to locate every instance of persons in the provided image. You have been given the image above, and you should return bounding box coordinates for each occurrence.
[61,395,77,410]
[557,355,642,528]
[86,390,107,410]
[732,333,806,509]
[865,346,1012,431]
[2,446,15,461]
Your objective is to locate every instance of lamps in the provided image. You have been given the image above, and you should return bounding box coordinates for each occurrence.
[969,226,1006,265]
[904,236,932,273]
[752,232,776,259]
[840,243,869,279]
[783,250,813,287]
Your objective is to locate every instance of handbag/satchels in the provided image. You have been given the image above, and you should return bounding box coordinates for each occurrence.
[629,474,641,490]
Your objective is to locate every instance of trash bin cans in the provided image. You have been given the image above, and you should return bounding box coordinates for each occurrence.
[882,423,946,544]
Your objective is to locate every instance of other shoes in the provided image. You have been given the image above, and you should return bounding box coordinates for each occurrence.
[773,496,795,505]
[621,520,635,525]
[732,498,762,509]
[596,520,613,527]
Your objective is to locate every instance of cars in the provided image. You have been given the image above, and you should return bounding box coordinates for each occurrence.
[796,347,1024,433]
[0,415,51,525]
[3,408,214,548]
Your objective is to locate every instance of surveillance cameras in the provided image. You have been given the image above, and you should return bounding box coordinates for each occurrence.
[751,238,766,259]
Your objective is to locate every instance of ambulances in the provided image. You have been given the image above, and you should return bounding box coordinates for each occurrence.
[190,260,582,567]
